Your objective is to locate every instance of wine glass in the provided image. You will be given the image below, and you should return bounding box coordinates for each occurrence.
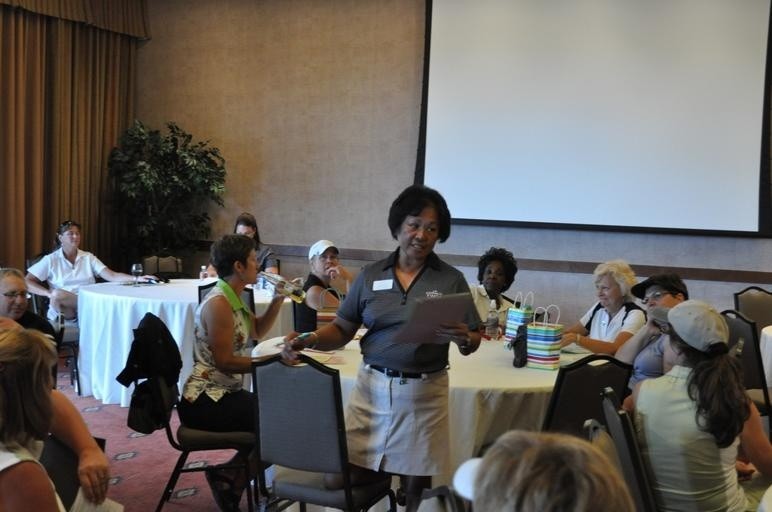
[131,263,143,287]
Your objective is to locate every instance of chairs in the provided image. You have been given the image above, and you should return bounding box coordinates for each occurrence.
[600,386,657,512]
[254,352,397,512]
[720,310,772,442]
[117,313,271,512]
[143,252,191,280]
[541,354,633,442]
[291,287,319,332]
[734,284,772,340]
[26,252,82,396]
[40,431,106,510]
[198,282,257,348]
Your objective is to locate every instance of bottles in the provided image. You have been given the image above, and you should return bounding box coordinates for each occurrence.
[485,299,499,341]
[200,265,207,284]
[259,271,307,305]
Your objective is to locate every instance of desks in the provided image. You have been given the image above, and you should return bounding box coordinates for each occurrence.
[79,278,295,409]
[250,330,611,493]
[758,325,772,389]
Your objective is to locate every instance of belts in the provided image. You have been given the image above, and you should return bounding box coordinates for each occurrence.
[369,365,447,379]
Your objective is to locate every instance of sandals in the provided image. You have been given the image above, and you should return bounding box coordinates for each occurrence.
[205,464,244,512]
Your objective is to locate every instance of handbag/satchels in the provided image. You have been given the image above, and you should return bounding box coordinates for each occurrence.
[317,305,345,350]
[504,308,534,343]
[127,378,173,434]
[526,321,561,371]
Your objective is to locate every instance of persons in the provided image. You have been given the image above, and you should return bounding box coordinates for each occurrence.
[180,213,277,510]
[468,246,518,331]
[2,267,109,511]
[284,184,485,511]
[472,430,636,511]
[303,239,354,334]
[558,261,771,511]
[23,220,159,330]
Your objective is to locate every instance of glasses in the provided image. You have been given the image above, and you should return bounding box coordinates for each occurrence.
[640,290,671,304]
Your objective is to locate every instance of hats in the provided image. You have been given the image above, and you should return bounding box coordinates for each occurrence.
[651,299,730,353]
[308,239,339,260]
[631,272,688,300]
